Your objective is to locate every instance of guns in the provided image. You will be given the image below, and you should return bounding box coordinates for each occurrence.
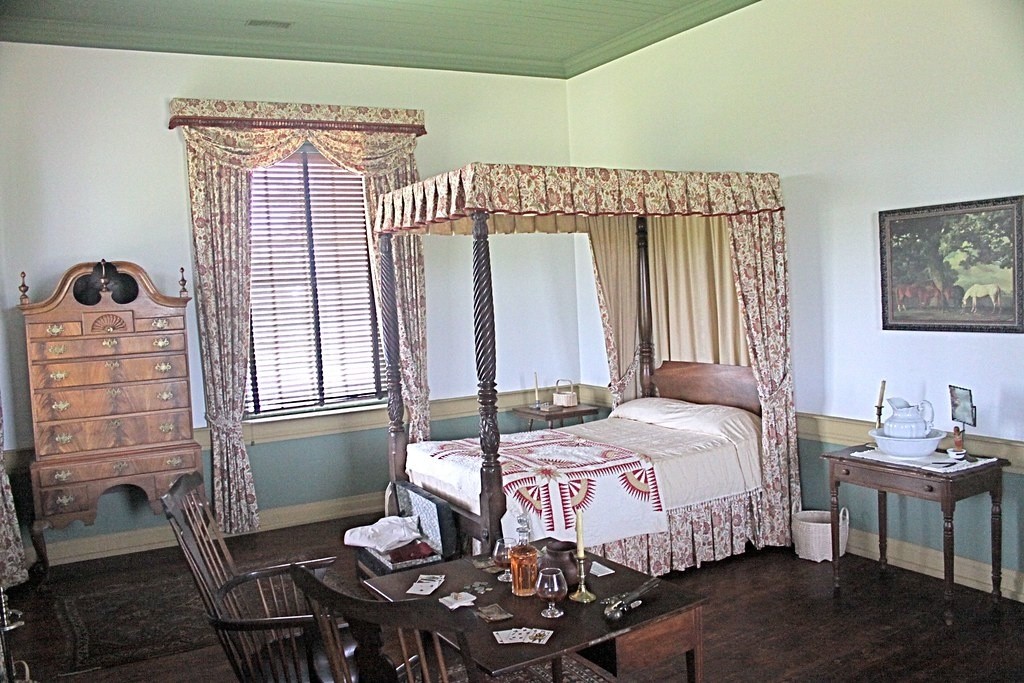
[599,577,662,622]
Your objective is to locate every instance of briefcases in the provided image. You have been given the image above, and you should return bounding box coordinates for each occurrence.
[354,478,459,602]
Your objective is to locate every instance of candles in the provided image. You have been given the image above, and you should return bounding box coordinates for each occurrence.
[576,506,585,558]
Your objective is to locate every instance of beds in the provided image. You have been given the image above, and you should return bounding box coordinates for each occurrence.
[374,160,802,577]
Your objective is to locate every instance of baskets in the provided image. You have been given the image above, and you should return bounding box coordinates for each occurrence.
[552,378,579,408]
[791,500,850,563]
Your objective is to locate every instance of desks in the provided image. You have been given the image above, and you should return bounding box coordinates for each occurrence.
[364,536,710,683]
[505,403,599,432]
[819,443,1011,626]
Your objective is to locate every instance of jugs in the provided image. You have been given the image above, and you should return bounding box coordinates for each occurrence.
[885,397,936,438]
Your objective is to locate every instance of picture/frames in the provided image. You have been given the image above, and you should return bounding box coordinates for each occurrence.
[878,195,1024,333]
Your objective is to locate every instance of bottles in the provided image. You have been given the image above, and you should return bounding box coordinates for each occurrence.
[510,527,540,596]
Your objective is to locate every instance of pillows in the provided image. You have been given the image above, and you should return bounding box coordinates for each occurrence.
[610,397,689,437]
[675,404,760,437]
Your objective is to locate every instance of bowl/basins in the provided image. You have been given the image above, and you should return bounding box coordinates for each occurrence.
[867,427,947,457]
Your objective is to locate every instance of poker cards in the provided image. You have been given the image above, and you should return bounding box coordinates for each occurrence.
[492,625,554,645]
[404,573,446,596]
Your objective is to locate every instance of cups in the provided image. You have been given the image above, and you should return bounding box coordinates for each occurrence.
[946,448,966,459]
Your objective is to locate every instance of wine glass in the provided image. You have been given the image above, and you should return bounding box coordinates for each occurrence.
[492,537,517,582]
[534,567,569,618]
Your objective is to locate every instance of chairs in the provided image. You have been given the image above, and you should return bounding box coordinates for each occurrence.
[161,470,359,683]
[289,561,479,683]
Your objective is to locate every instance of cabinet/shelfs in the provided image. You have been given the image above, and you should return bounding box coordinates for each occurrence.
[16,258,212,575]
